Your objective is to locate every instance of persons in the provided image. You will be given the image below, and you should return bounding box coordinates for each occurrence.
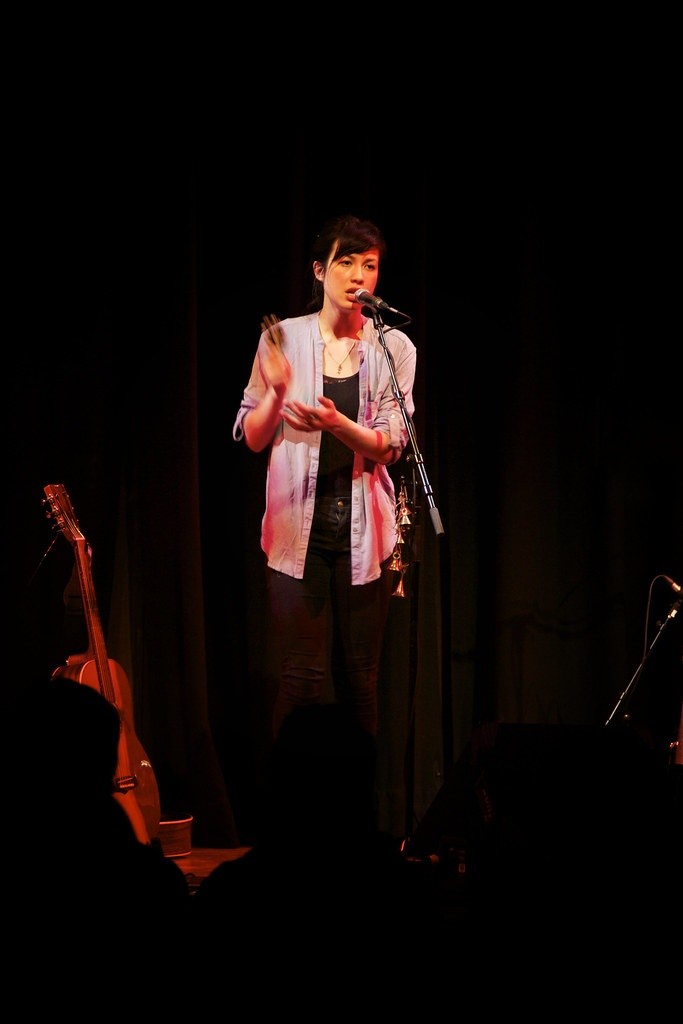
[1,674,683,1024]
[231,217,418,735]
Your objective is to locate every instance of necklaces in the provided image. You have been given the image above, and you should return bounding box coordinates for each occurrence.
[325,338,358,374]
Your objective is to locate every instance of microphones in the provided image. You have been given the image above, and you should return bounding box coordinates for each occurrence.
[354,288,389,310]
[664,576,683,599]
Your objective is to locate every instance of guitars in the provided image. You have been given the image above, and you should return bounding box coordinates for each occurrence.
[42,482,194,859]
